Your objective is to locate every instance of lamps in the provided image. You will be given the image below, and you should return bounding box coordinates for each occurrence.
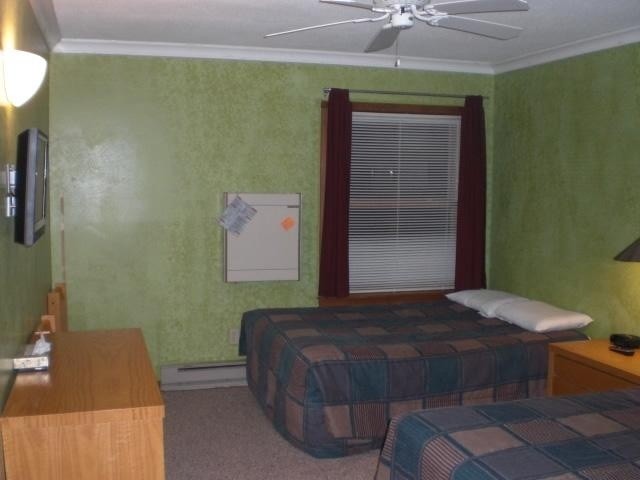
[1,43,49,108]
[612,232,640,264]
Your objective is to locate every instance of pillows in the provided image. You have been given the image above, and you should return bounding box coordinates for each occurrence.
[496,303,596,336]
[442,285,530,321]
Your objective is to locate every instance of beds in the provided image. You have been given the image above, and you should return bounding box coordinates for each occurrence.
[372,379,639,478]
[233,287,593,462]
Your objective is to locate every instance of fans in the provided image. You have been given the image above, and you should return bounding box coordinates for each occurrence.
[261,0,537,48]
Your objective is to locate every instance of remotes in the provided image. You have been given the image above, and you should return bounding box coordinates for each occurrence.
[609,345,635,355]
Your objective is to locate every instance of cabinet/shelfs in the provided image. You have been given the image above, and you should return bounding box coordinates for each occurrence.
[2,325,170,480]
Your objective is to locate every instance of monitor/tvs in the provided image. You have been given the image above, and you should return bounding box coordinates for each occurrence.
[15,126,50,247]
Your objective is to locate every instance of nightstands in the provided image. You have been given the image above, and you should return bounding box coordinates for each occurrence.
[540,334,640,397]
